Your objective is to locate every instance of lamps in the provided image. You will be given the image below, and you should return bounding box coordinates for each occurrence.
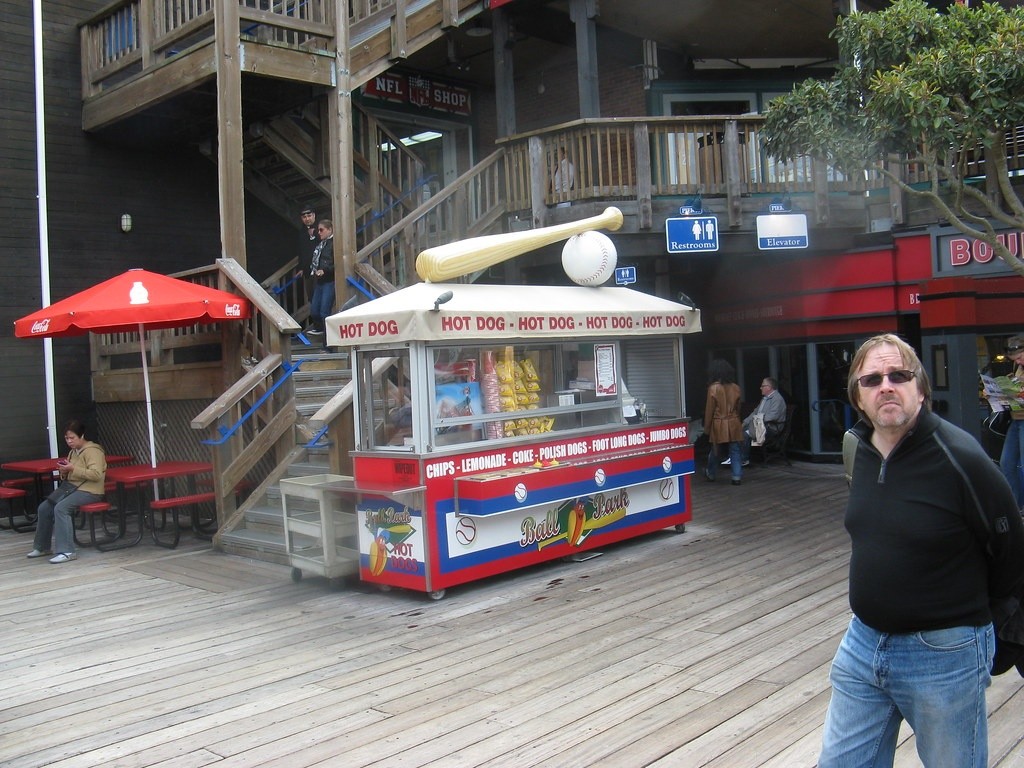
[771,191,792,210]
[117,214,132,233]
[684,188,701,211]
[429,291,453,311]
[677,291,697,313]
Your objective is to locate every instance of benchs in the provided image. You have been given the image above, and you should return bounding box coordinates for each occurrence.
[0,475,257,552]
[704,401,798,468]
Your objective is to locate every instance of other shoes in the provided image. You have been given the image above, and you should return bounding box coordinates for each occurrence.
[50,552,76,563]
[733,480,741,484]
[26,550,51,557]
[705,468,715,481]
[742,460,749,466]
[721,458,731,464]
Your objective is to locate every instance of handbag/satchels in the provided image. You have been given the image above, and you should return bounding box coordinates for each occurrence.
[47,481,78,504]
[981,405,1011,437]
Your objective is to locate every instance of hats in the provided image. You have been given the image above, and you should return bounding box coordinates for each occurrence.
[299,203,313,216]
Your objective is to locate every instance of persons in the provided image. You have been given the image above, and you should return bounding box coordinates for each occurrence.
[701,359,744,486]
[27,421,107,564]
[818,335,1024,768]
[721,377,787,466]
[778,358,846,447]
[548,146,574,209]
[297,204,335,353]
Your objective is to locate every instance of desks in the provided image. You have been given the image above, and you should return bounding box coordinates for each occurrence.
[106,461,214,540]
[2,456,135,526]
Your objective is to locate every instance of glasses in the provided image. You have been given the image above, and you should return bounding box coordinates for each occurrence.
[856,370,917,387]
[318,227,329,233]
[1003,346,1024,354]
[761,385,769,389]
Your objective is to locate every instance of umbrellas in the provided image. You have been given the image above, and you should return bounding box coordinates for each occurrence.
[15,267,255,500]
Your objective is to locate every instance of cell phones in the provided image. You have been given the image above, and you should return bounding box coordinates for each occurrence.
[58,461,67,465]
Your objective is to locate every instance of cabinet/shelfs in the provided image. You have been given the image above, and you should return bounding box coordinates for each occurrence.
[279,474,359,591]
